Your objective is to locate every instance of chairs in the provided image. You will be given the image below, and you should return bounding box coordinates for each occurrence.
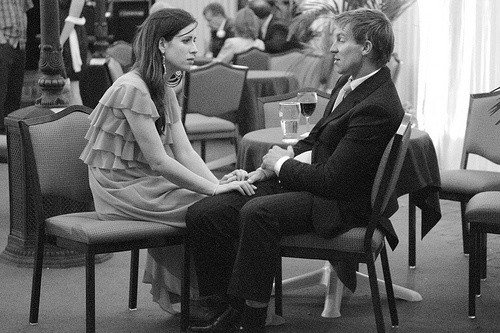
[267,49,324,88]
[408,90,500,269]
[19,105,190,333]
[272,112,411,333]
[465,191,500,319]
[233,46,270,71]
[181,61,249,162]
[256,88,331,129]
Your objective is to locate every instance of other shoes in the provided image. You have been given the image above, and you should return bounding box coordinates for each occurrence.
[267,313,285,325]
[193,299,267,333]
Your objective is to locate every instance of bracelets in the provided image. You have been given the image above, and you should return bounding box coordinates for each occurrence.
[212,184,219,196]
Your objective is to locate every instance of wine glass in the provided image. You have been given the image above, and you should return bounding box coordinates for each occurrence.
[297,92,317,133]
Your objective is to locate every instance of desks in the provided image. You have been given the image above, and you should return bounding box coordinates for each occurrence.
[222,71,299,132]
[236,125,442,317]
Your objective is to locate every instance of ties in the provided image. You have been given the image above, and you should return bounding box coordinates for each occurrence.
[331,81,352,112]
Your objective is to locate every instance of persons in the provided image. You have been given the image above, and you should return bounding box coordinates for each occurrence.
[79,7,285,325]
[248,0,304,53]
[60,0,87,106]
[215,8,265,60]
[188,7,404,333]
[203,2,234,59]
[0,0,34,135]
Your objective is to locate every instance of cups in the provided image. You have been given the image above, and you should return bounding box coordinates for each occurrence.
[279,102,301,135]
